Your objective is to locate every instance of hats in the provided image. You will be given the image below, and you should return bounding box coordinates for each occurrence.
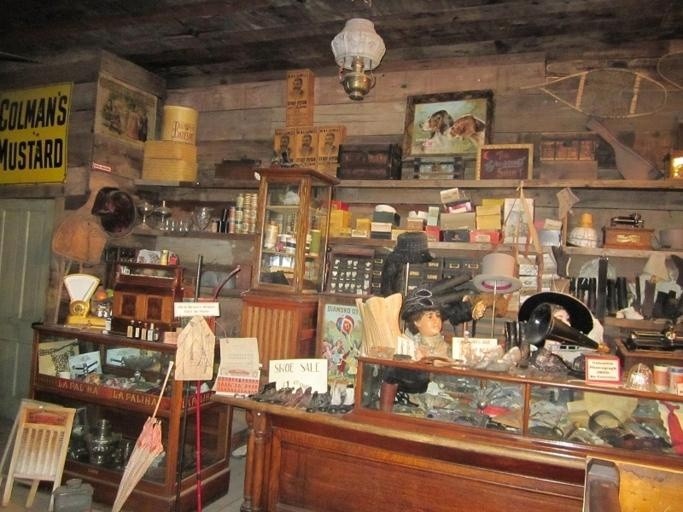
[386,230,434,264]
[471,252,523,295]
[517,290,593,349]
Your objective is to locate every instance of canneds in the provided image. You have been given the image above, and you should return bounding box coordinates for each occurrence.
[228,192,257,235]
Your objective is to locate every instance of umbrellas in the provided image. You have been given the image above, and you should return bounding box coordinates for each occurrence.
[105,359,173,512]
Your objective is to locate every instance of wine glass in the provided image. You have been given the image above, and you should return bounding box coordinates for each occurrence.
[155,201,173,230]
[136,204,154,230]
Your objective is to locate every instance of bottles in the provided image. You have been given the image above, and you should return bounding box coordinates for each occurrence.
[126,320,159,341]
[159,250,168,266]
[309,229,322,257]
[263,221,280,248]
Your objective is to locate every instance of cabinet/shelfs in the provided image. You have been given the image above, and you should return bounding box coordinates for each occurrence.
[31,321,232,512]
[325,177,683,258]
[250,167,342,297]
[132,178,270,239]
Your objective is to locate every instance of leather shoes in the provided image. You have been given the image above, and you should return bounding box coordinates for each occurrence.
[249,381,355,414]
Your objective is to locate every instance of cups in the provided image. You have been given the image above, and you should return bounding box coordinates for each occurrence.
[304,234,312,255]
[285,237,296,255]
[669,366,683,395]
[278,235,292,252]
[172,204,212,234]
[653,363,669,393]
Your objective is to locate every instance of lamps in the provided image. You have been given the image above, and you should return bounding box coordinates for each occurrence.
[332,0,387,99]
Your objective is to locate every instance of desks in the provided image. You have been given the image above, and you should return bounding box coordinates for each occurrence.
[211,355,683,511]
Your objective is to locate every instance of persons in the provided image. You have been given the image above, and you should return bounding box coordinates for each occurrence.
[322,132,337,155]
[289,77,305,101]
[98,93,149,144]
[276,134,291,155]
[298,134,313,156]
[399,283,455,361]
[547,302,575,332]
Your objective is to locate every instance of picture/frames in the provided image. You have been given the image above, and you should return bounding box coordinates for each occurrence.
[402,89,495,157]
[476,144,534,179]
